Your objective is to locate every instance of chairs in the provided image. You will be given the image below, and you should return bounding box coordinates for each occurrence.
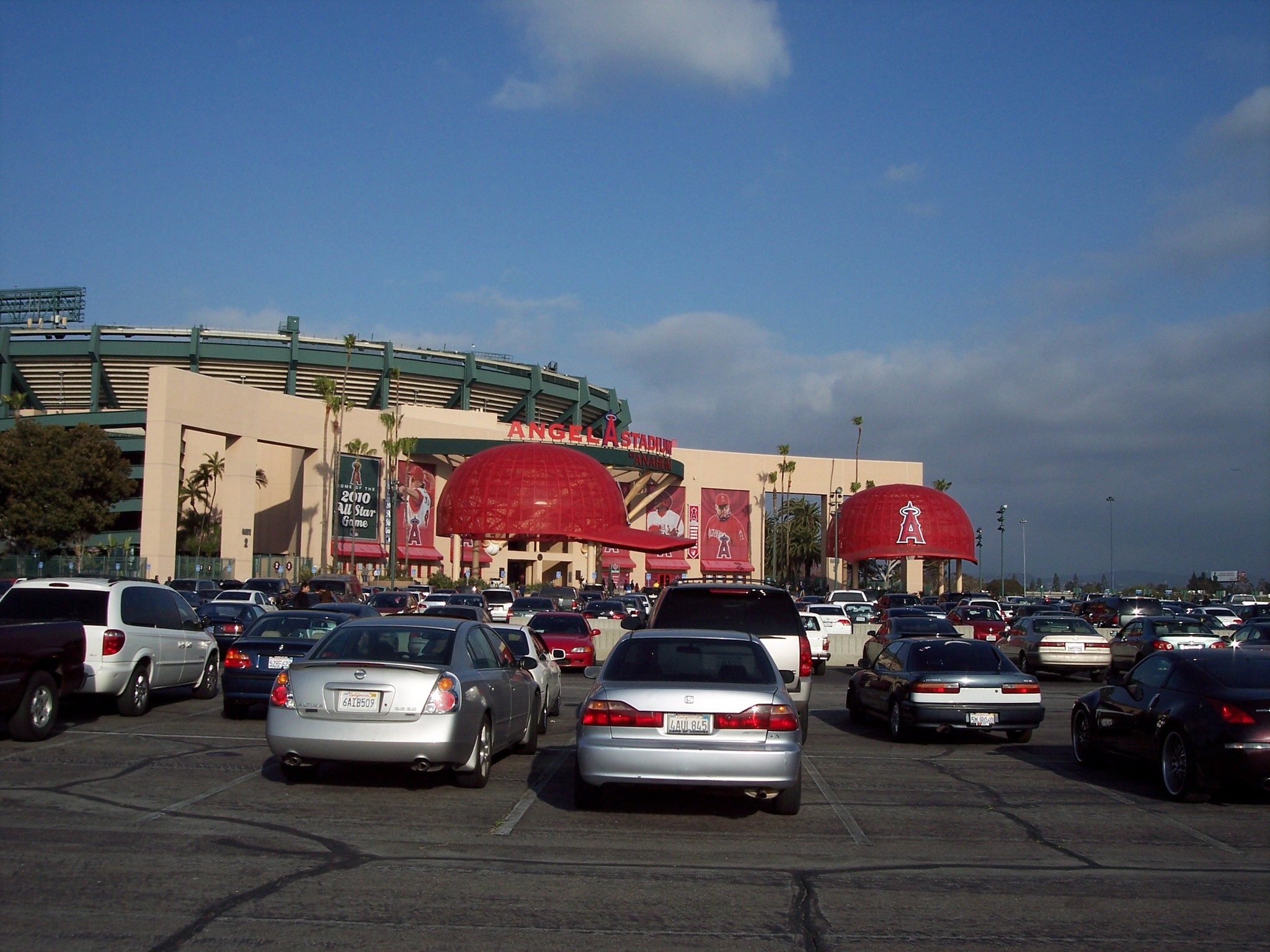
[718,665,747,683]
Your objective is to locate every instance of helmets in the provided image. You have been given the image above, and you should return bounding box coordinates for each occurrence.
[661,491,672,510]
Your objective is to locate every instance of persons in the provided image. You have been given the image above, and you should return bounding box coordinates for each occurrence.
[398,466,431,528]
[867,585,873,590]
[653,580,660,589]
[770,580,830,598]
[876,585,882,591]
[353,561,403,583]
[1190,594,1212,605]
[1178,597,1181,602]
[602,577,640,596]
[579,576,585,590]
[698,581,700,583]
[293,582,313,610]
[155,575,172,586]
[1254,603,1259,617]
[489,568,526,597]
[1213,572,1218,581]
[647,491,684,537]
[393,587,399,592]
[1038,595,1050,605]
[1161,597,1164,601]
[671,574,680,585]
[1059,596,1065,604]
[916,590,921,599]
[703,494,747,547]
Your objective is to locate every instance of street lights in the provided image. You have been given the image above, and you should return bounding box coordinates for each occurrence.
[996,504,1007,597]
[974,525,983,592]
[1106,495,1115,596]
[1019,518,1027,598]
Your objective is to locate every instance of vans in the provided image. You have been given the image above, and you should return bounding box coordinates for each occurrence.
[620,582,814,746]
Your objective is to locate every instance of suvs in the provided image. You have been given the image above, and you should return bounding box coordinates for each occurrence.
[0,575,221,717]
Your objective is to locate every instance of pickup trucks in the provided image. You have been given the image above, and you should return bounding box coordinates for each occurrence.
[0,620,89,743]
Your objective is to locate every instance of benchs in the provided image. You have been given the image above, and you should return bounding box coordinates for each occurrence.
[262,631,326,639]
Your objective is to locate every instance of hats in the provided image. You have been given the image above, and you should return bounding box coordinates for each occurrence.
[408,466,424,482]
[715,493,730,507]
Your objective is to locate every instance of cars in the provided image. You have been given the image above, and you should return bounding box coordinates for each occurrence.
[169,573,1270,684]
[483,622,566,717]
[220,609,362,719]
[1070,646,1270,803]
[574,632,804,816]
[264,614,547,789]
[845,635,1046,744]
